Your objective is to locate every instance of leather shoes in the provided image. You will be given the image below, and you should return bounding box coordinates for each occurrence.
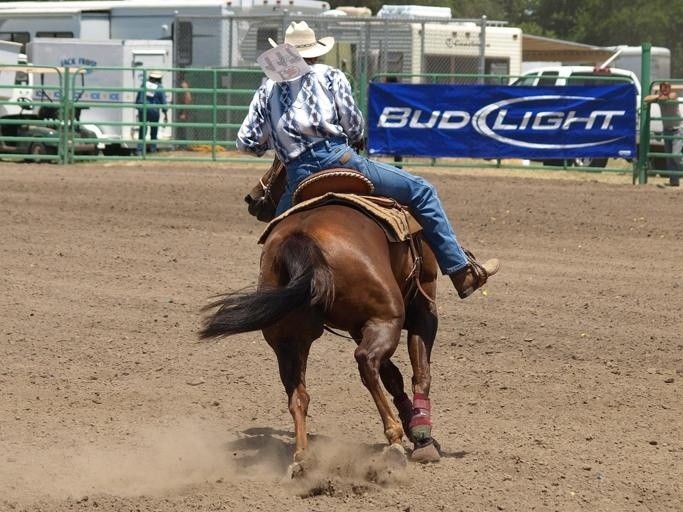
[449,258,500,299]
[148,70,162,79]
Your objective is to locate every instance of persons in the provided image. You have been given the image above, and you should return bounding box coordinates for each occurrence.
[237,22,500,299]
[133,72,169,141]
[643,81,683,186]
[176,73,192,150]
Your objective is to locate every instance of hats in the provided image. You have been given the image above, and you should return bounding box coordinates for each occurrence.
[267,20,336,59]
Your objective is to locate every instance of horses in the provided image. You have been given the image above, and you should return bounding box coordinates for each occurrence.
[194,150,440,480]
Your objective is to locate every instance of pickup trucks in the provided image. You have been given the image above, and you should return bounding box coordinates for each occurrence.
[501,66,683,174]
